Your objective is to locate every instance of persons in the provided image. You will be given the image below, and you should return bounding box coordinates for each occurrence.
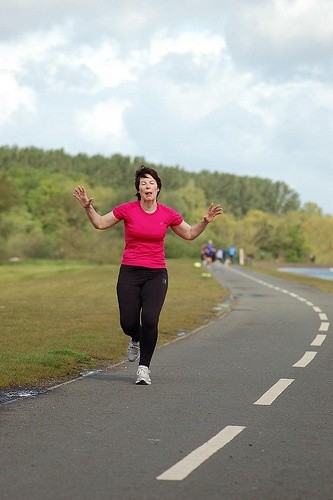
[200,237,236,266]
[71,164,223,386]
[309,253,317,263]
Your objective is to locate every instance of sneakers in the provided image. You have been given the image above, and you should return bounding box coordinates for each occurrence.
[136,364,152,385]
[125,338,140,363]
